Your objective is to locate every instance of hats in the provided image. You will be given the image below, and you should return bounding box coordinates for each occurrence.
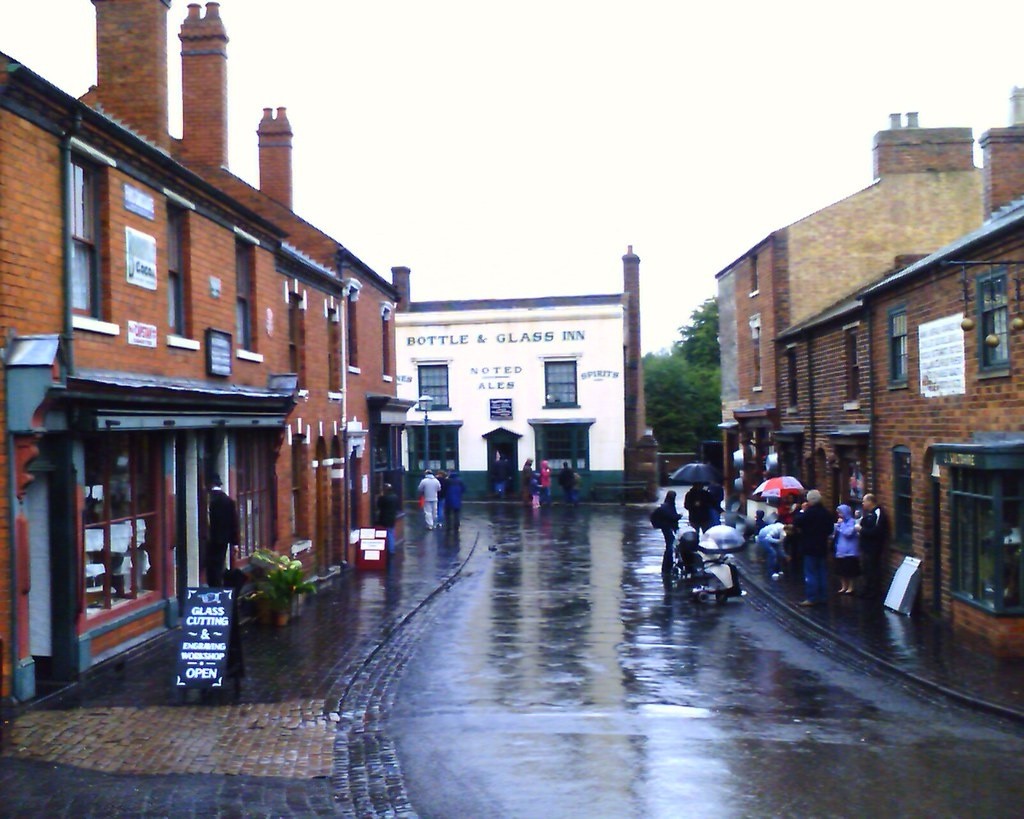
[207,474,223,486]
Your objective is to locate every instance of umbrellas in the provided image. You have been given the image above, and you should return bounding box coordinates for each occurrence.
[698,525,746,549]
[753,476,804,498]
[668,463,724,483]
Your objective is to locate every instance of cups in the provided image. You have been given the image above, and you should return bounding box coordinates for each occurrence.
[1011,528,1023,536]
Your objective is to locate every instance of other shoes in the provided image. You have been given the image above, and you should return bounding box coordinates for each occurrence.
[838,585,847,593]
[427,525,433,529]
[772,572,779,577]
[779,571,784,574]
[802,599,818,605]
[533,505,541,509]
[846,585,854,593]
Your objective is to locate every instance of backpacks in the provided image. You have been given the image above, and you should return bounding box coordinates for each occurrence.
[650,506,665,528]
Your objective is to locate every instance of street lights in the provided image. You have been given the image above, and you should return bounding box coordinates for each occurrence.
[418,391,434,476]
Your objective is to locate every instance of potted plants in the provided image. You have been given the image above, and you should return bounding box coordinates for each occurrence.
[243,540,317,625]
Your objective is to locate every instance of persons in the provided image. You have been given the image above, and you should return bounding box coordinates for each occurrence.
[205,471,241,588]
[434,470,447,527]
[749,489,834,608]
[521,459,533,503]
[417,470,441,530]
[660,490,683,575]
[529,471,543,509]
[539,460,551,505]
[377,483,399,555]
[558,462,582,508]
[684,482,725,532]
[831,493,888,596]
[442,468,466,531]
[494,454,514,498]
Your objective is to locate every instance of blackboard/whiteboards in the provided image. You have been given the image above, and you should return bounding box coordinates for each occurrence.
[174,586,245,688]
